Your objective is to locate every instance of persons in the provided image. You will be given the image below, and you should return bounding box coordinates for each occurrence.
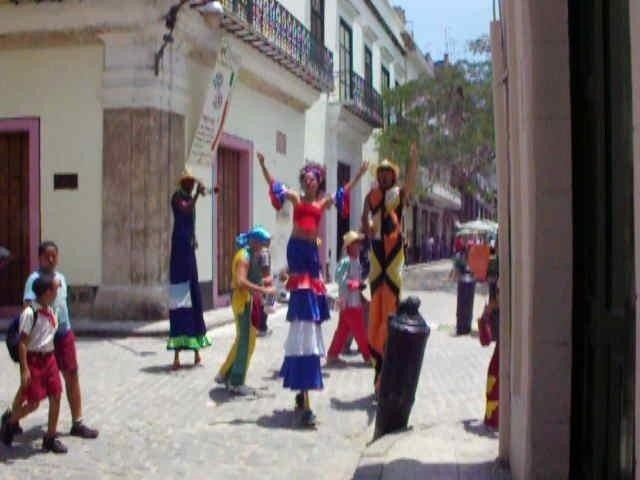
[255,152,369,425]
[424,232,435,264]
[476,297,499,438]
[448,231,499,298]
[326,141,417,402]
[0,241,98,452]
[214,224,278,396]
[168,176,206,370]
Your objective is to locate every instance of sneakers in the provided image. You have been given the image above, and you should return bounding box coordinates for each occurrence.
[41,433,67,453]
[0,410,23,447]
[70,421,99,439]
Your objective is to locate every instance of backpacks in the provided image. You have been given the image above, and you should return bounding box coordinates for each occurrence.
[7,303,38,362]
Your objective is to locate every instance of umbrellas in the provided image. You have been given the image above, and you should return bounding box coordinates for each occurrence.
[456,219,498,237]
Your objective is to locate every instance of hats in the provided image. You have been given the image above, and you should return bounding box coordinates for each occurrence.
[370,159,399,178]
[343,230,365,250]
[175,166,193,186]
